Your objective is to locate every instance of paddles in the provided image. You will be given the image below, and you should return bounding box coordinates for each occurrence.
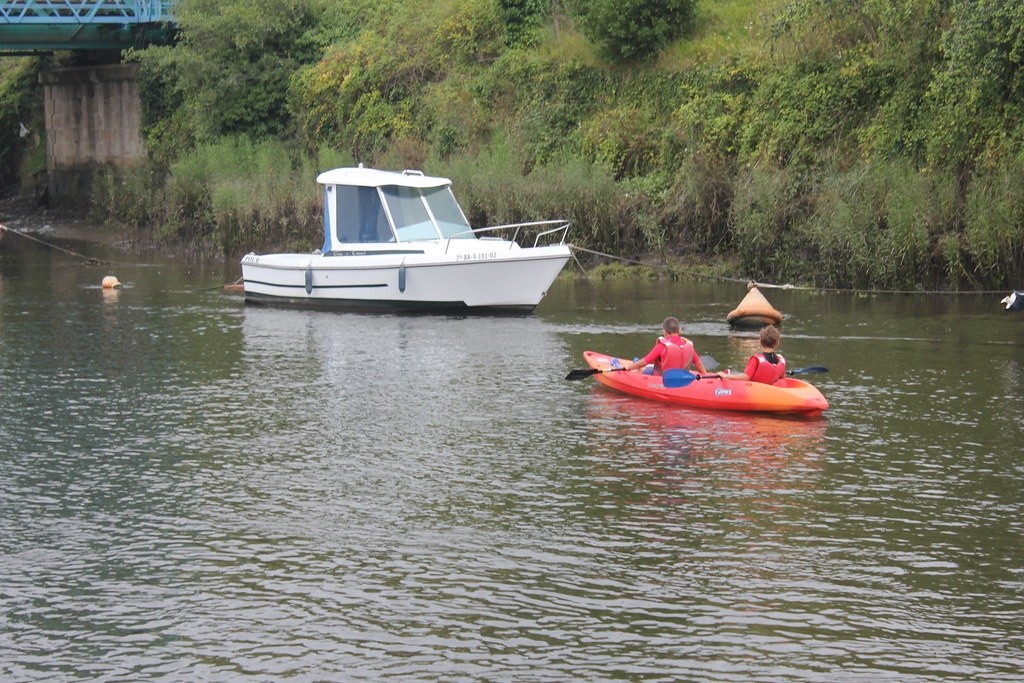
[662,366,830,388]
[565,355,718,381]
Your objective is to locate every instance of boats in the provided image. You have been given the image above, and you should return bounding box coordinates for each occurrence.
[583,350,830,419]
[240,163,573,316]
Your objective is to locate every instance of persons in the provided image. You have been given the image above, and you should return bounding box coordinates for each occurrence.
[623,317,707,375]
[717,325,787,385]
